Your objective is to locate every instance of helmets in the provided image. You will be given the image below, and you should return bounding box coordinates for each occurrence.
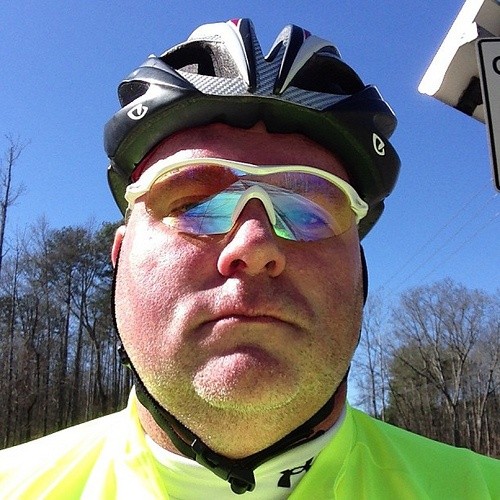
[104,18,402,242]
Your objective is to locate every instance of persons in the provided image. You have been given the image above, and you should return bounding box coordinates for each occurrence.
[0,19,500,499]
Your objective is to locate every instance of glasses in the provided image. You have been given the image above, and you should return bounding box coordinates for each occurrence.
[125,158,368,241]
